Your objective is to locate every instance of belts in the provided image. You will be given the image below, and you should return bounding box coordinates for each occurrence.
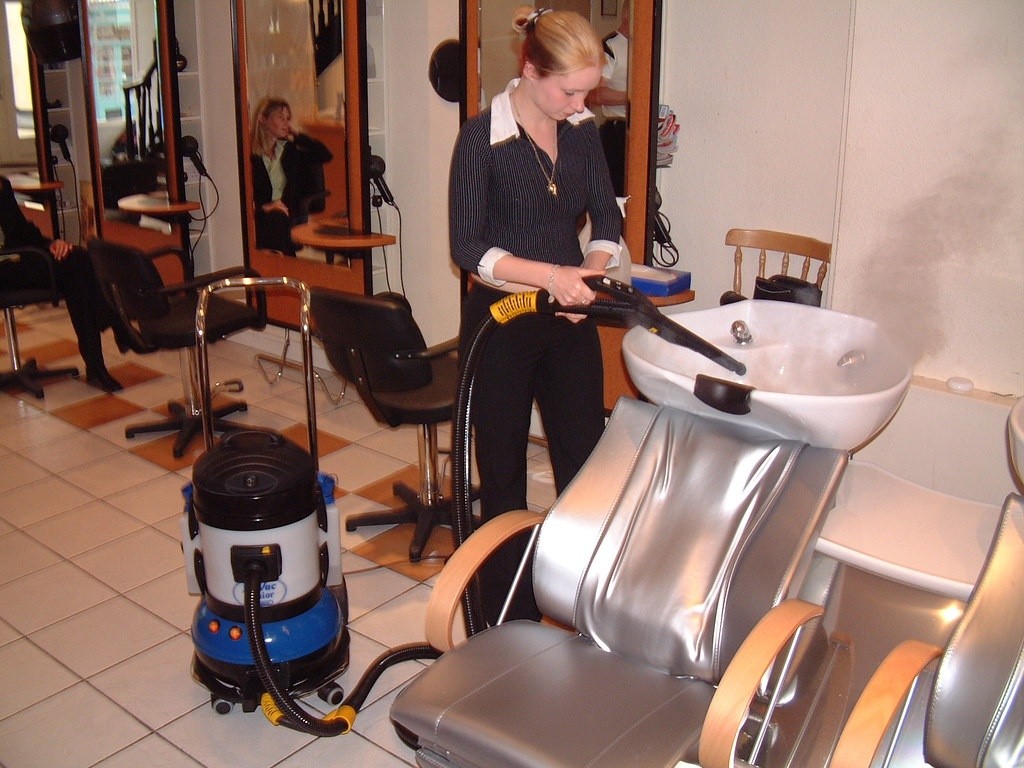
[604,119,626,127]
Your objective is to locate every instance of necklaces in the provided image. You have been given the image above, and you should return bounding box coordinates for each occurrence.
[512,93,556,196]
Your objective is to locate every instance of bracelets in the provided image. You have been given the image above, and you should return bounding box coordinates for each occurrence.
[548,264,559,303]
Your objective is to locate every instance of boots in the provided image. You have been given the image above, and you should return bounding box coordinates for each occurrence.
[77,336,125,392]
[102,279,159,354]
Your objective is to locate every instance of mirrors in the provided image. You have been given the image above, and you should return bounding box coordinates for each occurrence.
[0,0,61,241]
[230,0,373,335]
[458,0,662,419]
[82,0,191,285]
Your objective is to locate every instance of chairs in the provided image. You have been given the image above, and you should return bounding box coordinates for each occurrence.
[829,490,1024,768]
[293,165,331,252]
[0,245,79,399]
[308,284,481,564]
[86,236,269,459]
[724,226,832,297]
[389,399,850,768]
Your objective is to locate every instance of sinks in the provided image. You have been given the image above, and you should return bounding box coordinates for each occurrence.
[622,296,916,452]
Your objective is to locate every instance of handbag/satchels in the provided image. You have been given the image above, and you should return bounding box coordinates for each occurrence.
[753,275,822,307]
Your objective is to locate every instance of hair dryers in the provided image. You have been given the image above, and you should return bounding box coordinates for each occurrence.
[369,154,395,205]
[181,135,209,177]
[49,122,71,161]
[653,190,671,245]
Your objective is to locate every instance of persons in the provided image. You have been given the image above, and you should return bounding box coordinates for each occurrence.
[592,0,630,236]
[251,96,332,257]
[0,175,157,389]
[450,5,620,630]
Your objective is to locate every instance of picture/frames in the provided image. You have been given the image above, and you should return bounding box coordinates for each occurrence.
[601,0,618,17]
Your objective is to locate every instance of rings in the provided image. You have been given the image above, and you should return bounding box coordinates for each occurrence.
[581,298,587,305]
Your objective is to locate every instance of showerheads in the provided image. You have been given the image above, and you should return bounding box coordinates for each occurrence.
[534,272,747,378]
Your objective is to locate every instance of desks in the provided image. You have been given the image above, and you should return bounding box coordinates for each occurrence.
[7,170,65,192]
[116,193,201,224]
[290,218,396,260]
[596,290,696,308]
